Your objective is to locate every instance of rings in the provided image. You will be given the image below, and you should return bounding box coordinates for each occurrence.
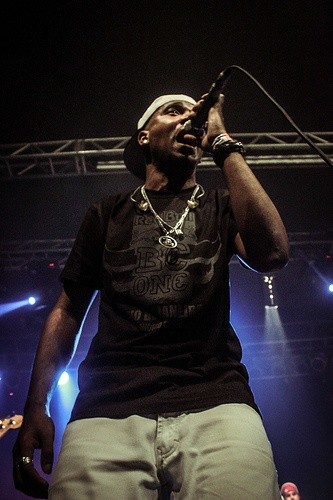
[17,457,34,467]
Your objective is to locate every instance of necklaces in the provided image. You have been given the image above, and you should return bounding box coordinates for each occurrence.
[130,184,205,249]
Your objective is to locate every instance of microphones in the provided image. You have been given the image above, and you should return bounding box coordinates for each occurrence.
[183,66,236,139]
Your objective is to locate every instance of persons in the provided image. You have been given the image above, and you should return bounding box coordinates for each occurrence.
[280,482,300,500]
[11,94,291,500]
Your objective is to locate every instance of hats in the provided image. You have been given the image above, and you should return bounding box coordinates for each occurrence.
[123,86,208,180]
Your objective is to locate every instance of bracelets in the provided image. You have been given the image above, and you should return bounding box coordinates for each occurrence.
[211,141,247,168]
